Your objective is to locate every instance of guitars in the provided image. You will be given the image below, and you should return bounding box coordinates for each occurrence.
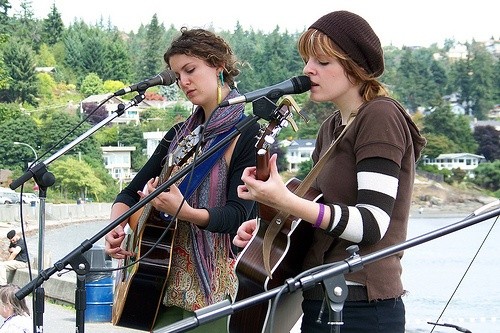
[227,95,326,333]
[110,125,205,332]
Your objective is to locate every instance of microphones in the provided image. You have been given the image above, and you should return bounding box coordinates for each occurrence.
[114,70,177,96]
[219,75,311,107]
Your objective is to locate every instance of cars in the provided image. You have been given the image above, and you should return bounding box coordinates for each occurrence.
[18,193,40,204]
[77,198,95,204]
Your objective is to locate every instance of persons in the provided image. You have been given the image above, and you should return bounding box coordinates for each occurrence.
[32,182,39,197]
[0,284,34,333]
[0,229,28,286]
[105,26,262,333]
[77,197,84,204]
[232,9,428,333]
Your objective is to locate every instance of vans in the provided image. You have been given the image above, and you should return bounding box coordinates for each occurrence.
[0,188,18,204]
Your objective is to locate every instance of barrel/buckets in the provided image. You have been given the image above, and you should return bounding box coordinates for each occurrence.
[81,247,112,322]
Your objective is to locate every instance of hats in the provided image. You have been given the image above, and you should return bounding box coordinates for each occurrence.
[309,10,385,79]
